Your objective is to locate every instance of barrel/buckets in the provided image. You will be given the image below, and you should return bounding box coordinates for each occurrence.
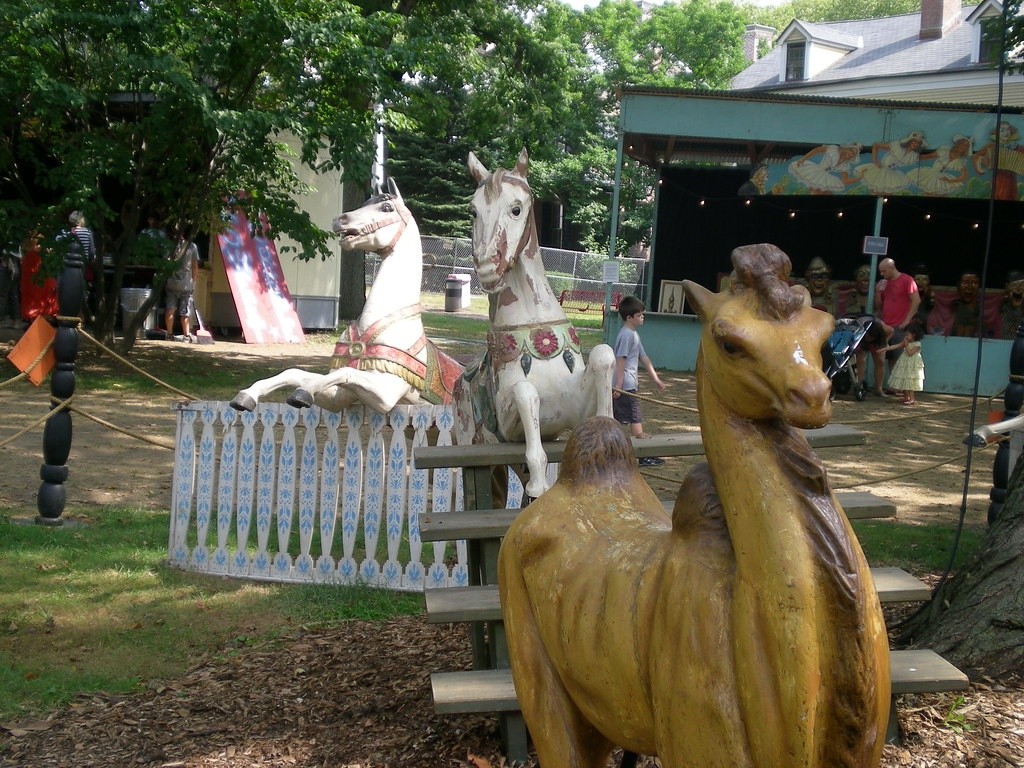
[121,288,155,338]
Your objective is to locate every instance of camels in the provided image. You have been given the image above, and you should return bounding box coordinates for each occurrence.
[494,242,894,768]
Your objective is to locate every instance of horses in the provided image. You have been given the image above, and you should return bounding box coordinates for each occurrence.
[227,175,469,420]
[454,145,618,504]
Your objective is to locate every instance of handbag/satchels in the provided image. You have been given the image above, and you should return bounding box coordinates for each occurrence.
[84,258,102,281]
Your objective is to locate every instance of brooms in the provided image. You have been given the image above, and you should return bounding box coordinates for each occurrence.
[193,301,215,344]
[145,309,168,340]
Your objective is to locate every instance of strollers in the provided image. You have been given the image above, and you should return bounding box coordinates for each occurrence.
[821,314,873,402]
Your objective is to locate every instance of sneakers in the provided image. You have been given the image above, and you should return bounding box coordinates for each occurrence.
[638,456,666,465]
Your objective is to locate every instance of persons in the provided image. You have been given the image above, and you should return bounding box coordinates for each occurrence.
[54,210,94,261]
[876,323,925,405]
[793,255,936,335]
[981,271,1024,340]
[141,207,167,239]
[0,242,22,329]
[874,257,922,394]
[853,313,895,398]
[612,295,672,466]
[927,272,981,338]
[164,217,200,342]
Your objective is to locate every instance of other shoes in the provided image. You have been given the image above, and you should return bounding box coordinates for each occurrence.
[164,334,181,341]
[182,333,197,343]
[897,398,914,404]
[874,391,888,398]
[9,323,20,329]
[0,321,10,328]
